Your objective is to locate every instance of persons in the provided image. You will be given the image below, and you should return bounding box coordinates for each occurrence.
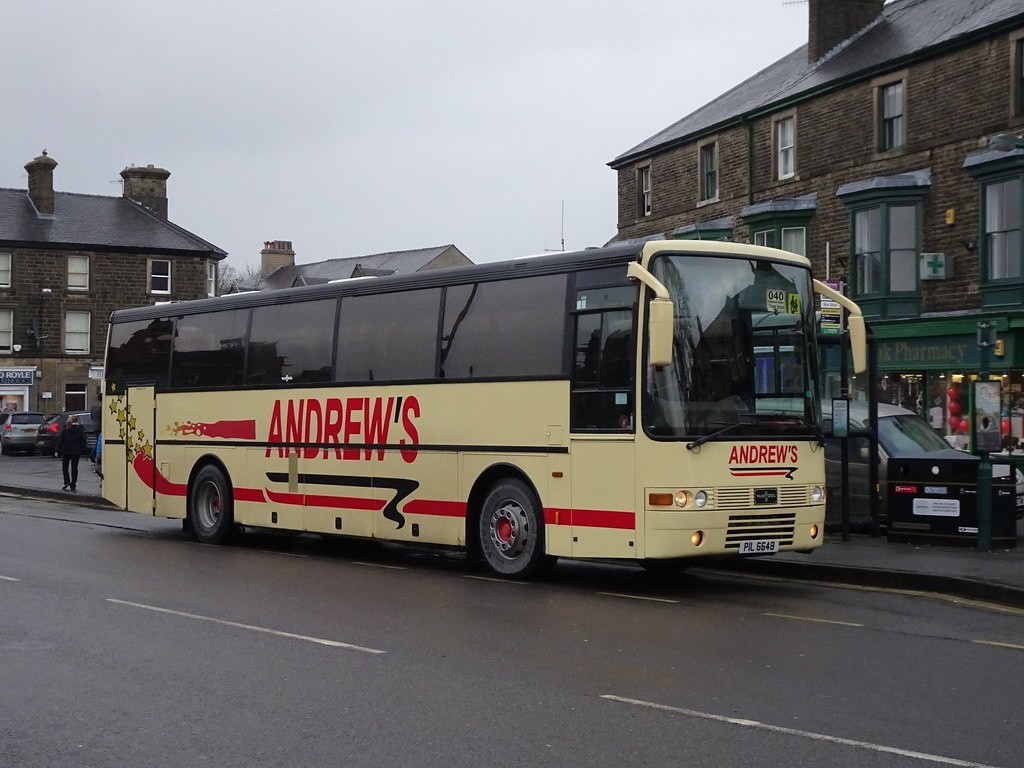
[61,414,86,492]
[10,403,17,412]
[90,393,102,465]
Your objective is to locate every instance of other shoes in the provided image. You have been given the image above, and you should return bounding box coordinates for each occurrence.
[99,478,102,488]
[62,482,70,489]
[70,483,76,490]
[89,454,96,463]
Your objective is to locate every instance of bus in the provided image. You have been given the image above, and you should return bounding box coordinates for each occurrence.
[100,239,869,584]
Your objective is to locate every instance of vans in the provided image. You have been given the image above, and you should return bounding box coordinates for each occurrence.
[755,399,1024,524]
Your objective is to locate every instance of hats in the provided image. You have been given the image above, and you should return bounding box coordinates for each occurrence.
[97,393,102,401]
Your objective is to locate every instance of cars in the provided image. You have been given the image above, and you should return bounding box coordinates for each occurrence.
[0,411,47,455]
[36,411,97,458]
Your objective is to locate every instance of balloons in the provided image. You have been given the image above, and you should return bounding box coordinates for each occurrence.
[948,401,960,414]
[948,386,959,398]
[1001,410,1010,434]
[949,416,969,432]
